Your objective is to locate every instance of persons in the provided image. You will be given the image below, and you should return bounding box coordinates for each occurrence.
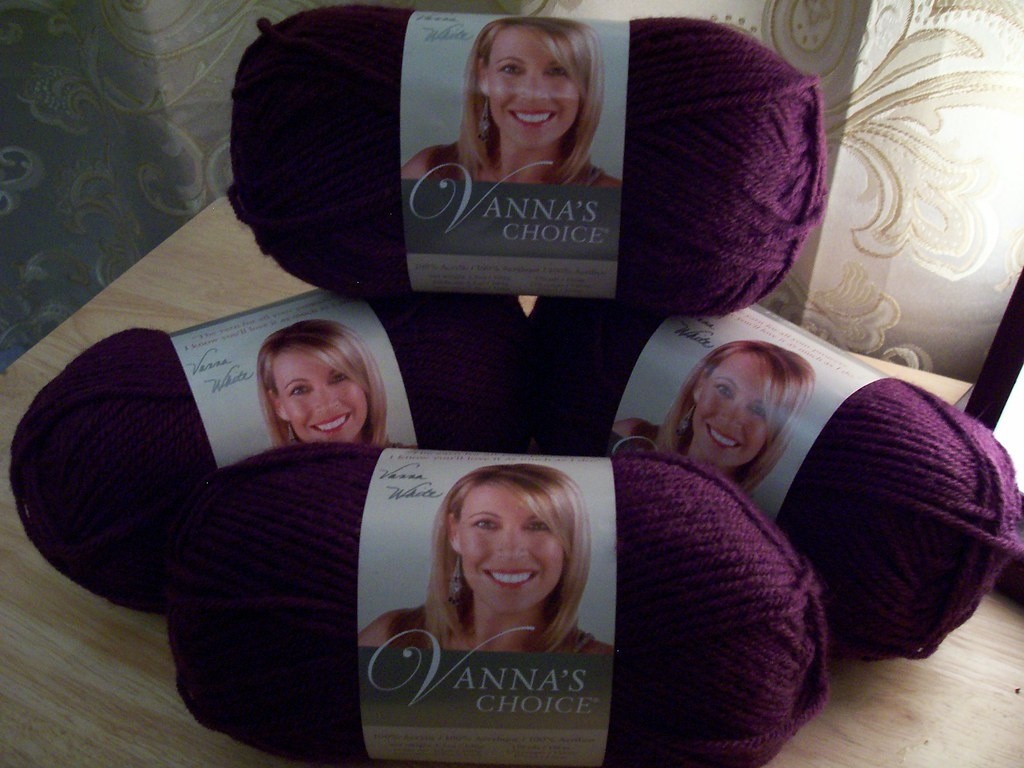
[612,340,817,494]
[402,17,621,185]
[256,318,418,448]
[358,464,614,655]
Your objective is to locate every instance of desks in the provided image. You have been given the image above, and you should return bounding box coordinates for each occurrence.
[1,194,1024,768]
